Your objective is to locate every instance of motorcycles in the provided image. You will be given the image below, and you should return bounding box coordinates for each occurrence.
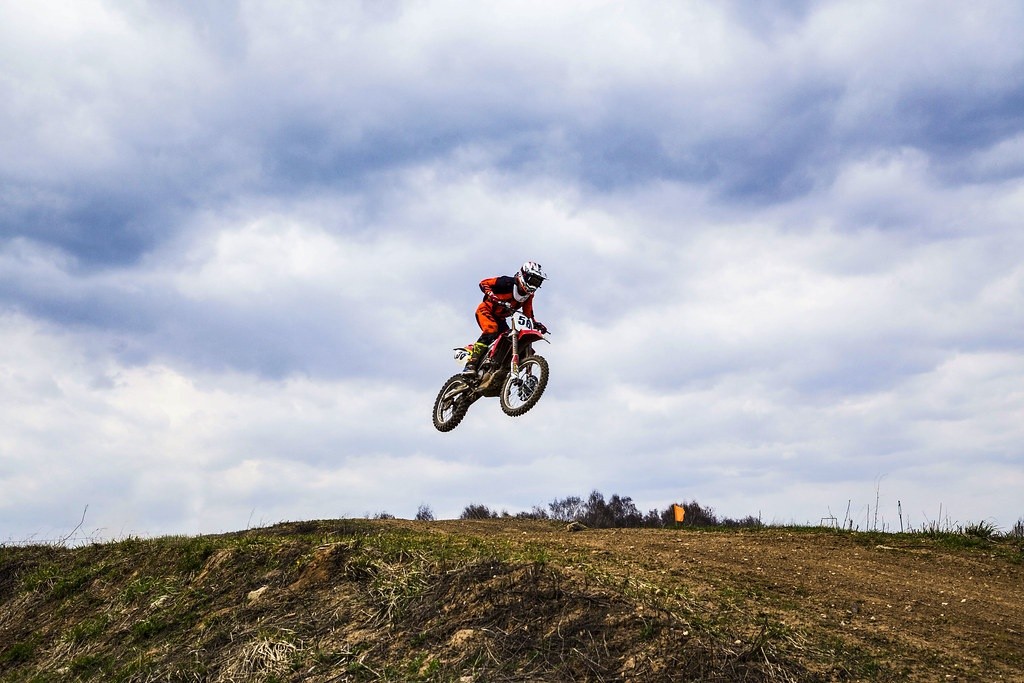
[432,299,552,433]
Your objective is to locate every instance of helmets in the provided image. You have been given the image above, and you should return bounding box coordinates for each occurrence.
[515,262,548,296]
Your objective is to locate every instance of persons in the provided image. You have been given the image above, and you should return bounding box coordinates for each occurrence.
[462,261,547,376]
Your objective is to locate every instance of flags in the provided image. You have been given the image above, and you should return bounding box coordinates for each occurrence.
[676,507,685,522]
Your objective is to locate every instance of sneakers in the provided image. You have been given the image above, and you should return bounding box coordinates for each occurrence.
[462,364,476,375]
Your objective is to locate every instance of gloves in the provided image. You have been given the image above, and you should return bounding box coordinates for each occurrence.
[534,322,547,334]
[488,296,499,305]
